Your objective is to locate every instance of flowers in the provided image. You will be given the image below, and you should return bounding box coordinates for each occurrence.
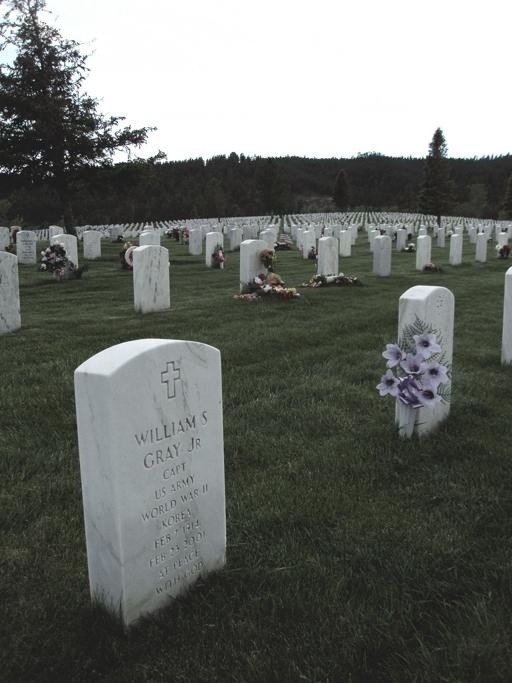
[308,246,318,259]
[167,226,190,242]
[260,249,277,270]
[212,243,225,263]
[254,272,300,296]
[39,241,69,277]
[499,245,511,259]
[376,312,458,409]
[119,242,139,269]
[301,273,358,287]
[408,243,415,247]
